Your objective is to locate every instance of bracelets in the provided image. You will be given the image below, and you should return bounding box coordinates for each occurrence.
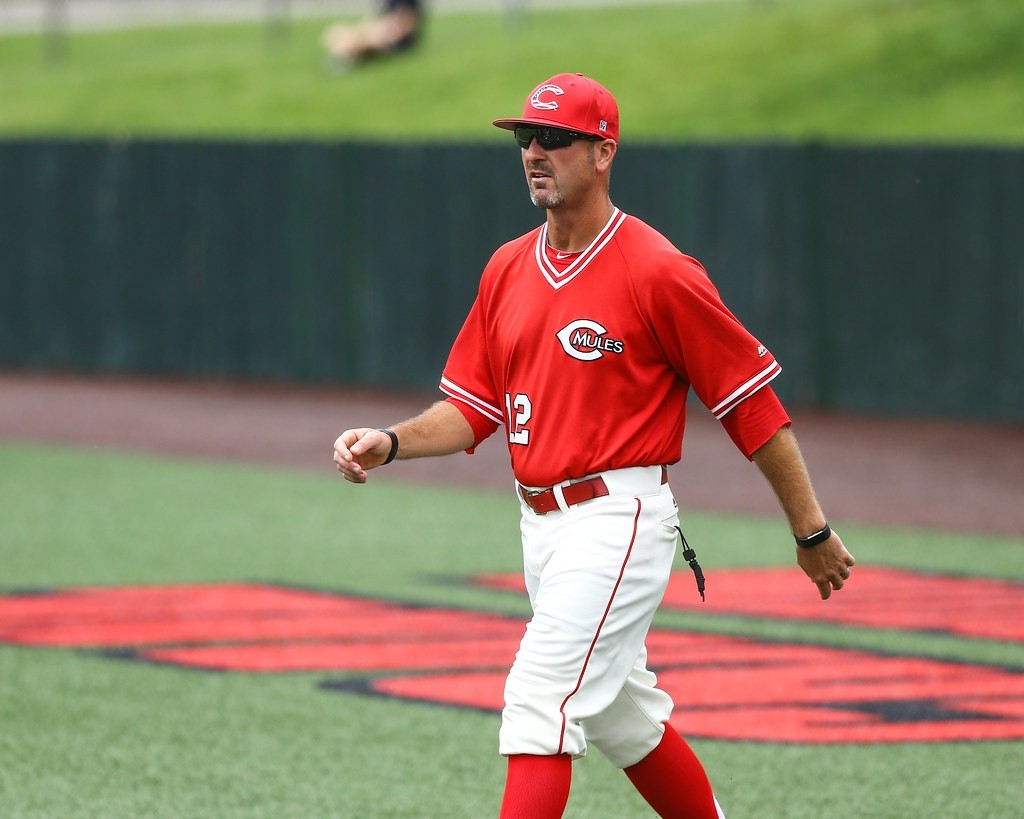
[377,426,398,464]
[794,522,832,547]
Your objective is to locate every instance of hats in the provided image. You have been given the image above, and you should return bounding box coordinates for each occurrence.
[493,73,619,144]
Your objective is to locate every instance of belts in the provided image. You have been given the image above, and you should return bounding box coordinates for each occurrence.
[518,463,669,515]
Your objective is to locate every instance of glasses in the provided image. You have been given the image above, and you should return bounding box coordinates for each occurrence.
[514,122,601,150]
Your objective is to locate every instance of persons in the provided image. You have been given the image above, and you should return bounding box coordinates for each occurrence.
[332,75,857,819]
[323,0,422,64]
